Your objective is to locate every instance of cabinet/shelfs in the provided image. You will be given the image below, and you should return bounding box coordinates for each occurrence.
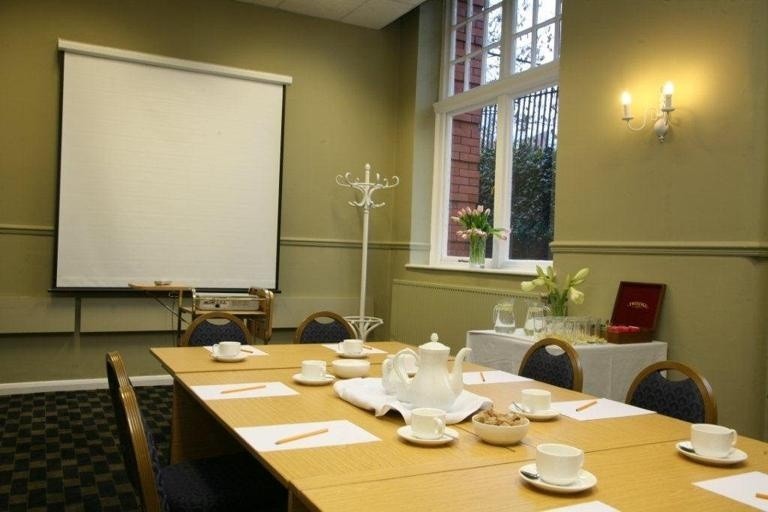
[177,287,274,343]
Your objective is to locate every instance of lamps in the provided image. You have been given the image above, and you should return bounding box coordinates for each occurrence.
[621,82,674,144]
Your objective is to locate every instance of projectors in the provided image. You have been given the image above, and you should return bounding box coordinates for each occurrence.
[194,293,260,310]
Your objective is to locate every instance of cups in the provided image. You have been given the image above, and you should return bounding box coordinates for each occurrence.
[338,339,364,353]
[534,316,609,345]
[691,423,738,458]
[301,360,327,380]
[536,444,585,486]
[520,390,552,413]
[212,342,241,357]
[399,354,416,370]
[411,408,446,440]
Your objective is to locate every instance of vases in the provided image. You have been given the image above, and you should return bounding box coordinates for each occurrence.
[470,240,486,269]
[451,204,511,243]
[551,305,567,321]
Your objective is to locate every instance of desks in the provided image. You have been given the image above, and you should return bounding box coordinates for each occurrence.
[466,328,667,404]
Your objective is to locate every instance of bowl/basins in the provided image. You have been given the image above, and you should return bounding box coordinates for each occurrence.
[472,413,530,446]
[332,359,371,379]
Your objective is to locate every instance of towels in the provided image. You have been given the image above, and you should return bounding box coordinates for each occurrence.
[333,377,494,424]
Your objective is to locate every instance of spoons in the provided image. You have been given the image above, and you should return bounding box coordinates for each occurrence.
[520,470,540,479]
[679,443,694,452]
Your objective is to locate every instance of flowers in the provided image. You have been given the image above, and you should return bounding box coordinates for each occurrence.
[520,264,589,305]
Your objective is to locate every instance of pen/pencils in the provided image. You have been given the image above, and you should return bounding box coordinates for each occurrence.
[274,426,331,447]
[575,399,599,413]
[363,342,374,353]
[756,491,768,501]
[239,348,255,354]
[219,385,267,395]
[478,371,487,386]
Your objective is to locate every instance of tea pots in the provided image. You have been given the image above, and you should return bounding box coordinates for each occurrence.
[523,299,554,338]
[492,298,517,335]
[393,333,472,409]
[381,354,409,396]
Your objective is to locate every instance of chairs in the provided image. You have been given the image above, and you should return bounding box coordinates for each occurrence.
[625,360,718,424]
[292,311,358,344]
[518,337,584,393]
[179,312,252,347]
[104,350,288,512]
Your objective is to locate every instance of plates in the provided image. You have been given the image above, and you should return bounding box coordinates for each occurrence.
[338,352,368,358]
[293,373,336,385]
[519,410,559,420]
[677,441,747,465]
[397,424,459,445]
[518,463,598,494]
[212,353,247,363]
